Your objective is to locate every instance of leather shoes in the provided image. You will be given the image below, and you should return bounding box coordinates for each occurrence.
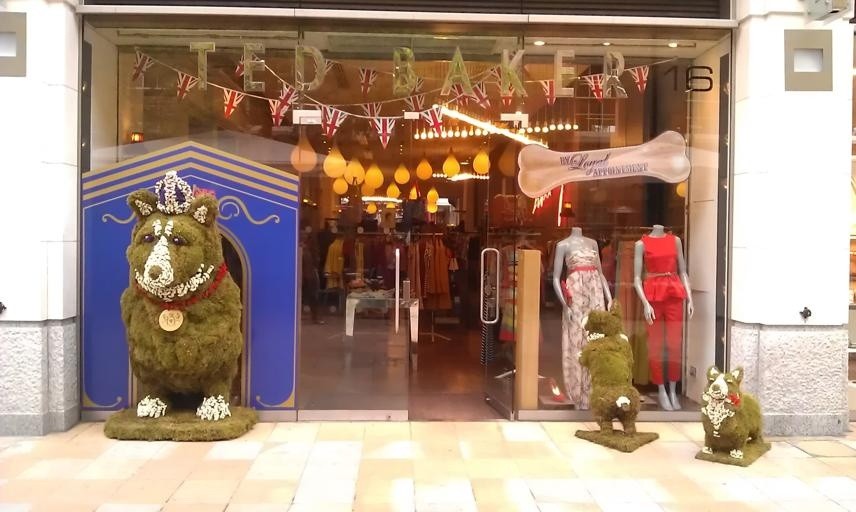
[549,376,565,402]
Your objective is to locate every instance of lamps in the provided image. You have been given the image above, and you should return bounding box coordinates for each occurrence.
[130,130,144,144]
[676,182,687,198]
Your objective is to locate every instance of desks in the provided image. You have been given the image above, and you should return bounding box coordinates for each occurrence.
[344,291,420,342]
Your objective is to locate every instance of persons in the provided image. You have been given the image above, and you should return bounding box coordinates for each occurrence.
[634,225,694,411]
[298,212,464,326]
[552,227,613,411]
